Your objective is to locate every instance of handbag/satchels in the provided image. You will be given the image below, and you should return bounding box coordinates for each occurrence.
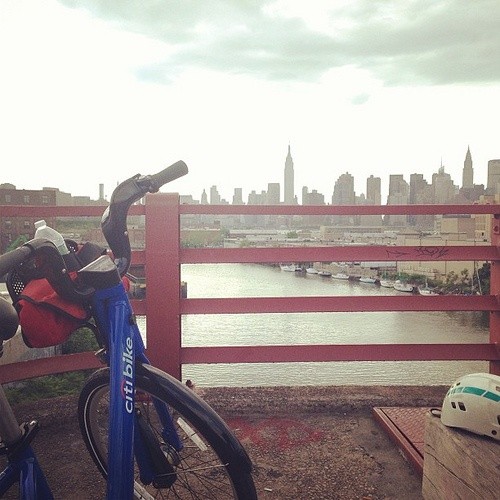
[15,244,129,349]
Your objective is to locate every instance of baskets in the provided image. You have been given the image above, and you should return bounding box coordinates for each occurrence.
[7,239,75,300]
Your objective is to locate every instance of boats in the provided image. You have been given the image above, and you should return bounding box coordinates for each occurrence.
[279,262,441,297]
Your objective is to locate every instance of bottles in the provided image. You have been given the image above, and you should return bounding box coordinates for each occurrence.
[31,219,68,254]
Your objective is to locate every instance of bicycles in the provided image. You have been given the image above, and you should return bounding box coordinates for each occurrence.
[0,157,258,500]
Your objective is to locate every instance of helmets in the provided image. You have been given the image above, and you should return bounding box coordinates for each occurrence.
[441,372,500,443]
